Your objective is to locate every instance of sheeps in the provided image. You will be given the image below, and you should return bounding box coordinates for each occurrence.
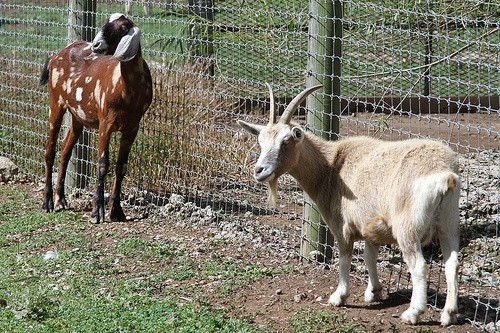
[39,12,153,224]
[236,82,463,326]
